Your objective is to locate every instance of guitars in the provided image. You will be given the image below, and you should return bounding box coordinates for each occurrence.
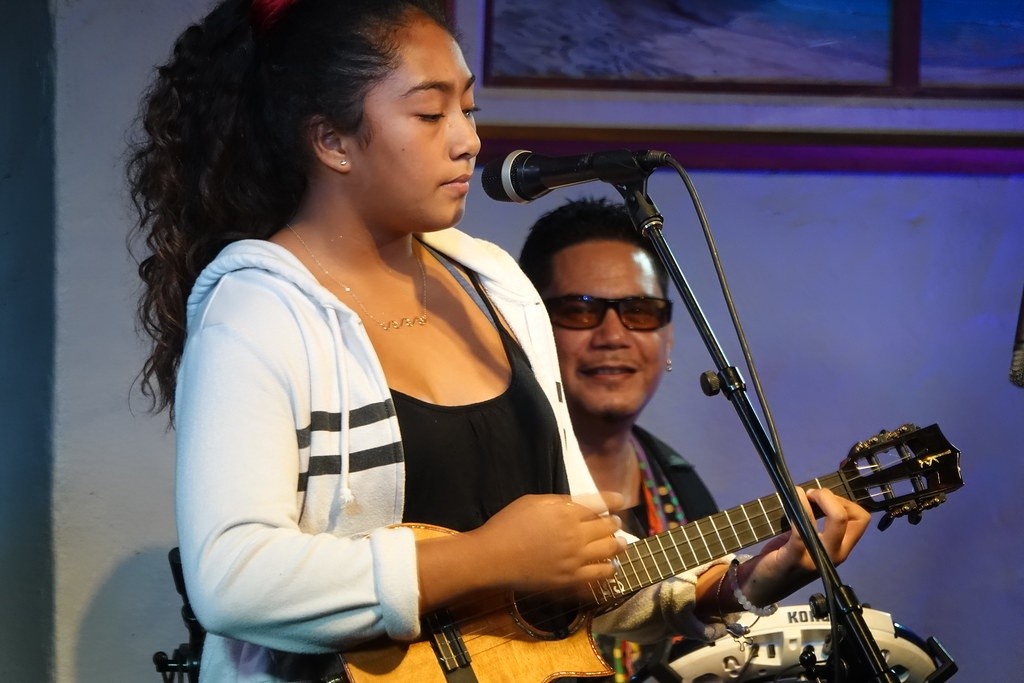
[315,421,965,683]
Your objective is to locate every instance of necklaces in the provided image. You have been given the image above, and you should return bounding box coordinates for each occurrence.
[285,220,427,330]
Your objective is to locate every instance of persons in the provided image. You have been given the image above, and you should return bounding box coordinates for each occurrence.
[518,196,722,683]
[123,0,872,683]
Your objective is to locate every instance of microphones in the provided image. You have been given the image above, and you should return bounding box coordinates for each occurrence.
[482,149,670,205]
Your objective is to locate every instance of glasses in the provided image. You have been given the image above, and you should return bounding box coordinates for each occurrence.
[541,295,674,332]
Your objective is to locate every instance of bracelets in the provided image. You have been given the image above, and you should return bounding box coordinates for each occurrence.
[727,554,780,617]
[715,567,761,652]
